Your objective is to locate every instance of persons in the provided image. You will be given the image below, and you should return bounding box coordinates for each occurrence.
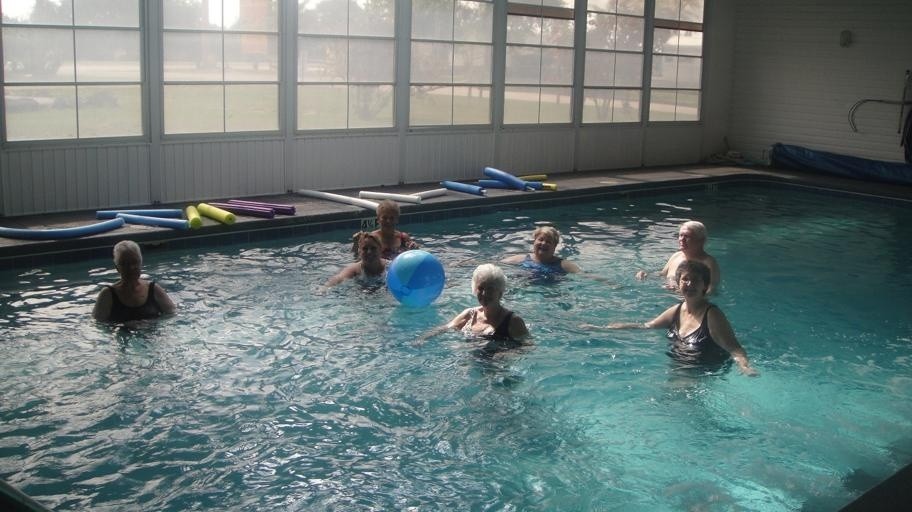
[317,231,394,297]
[449,226,623,311]
[368,199,420,261]
[89,239,177,330]
[635,220,721,295]
[575,260,763,380]
[413,261,536,361]
